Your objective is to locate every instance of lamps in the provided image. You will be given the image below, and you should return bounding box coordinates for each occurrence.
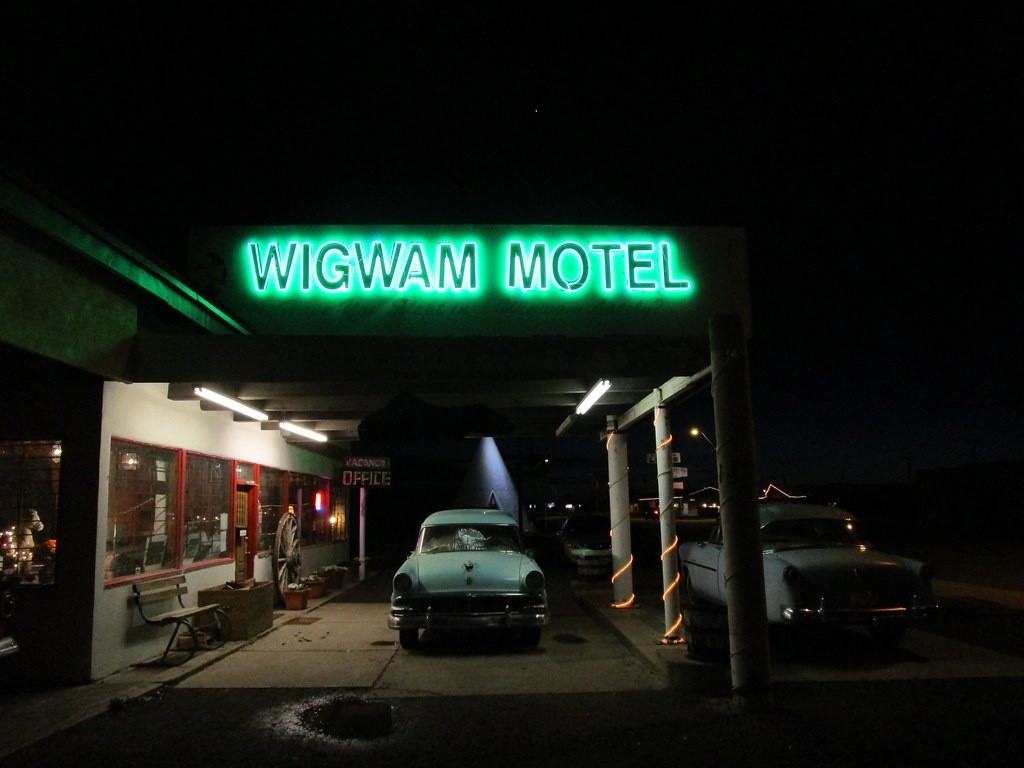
[280,420,328,442]
[555,415,576,436]
[192,382,269,422]
[575,378,614,415]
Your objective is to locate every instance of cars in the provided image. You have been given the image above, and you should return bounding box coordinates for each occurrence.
[387,508,550,653]
[555,516,611,576]
[677,503,944,651]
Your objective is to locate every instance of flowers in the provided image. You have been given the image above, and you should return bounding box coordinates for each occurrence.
[36,539,56,562]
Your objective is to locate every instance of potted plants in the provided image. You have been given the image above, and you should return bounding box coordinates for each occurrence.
[299,571,326,599]
[321,564,349,589]
[284,583,312,610]
[338,558,361,583]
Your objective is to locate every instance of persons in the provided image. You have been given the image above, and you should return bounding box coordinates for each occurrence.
[104,537,135,579]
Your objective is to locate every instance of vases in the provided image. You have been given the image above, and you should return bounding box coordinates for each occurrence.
[38,559,54,584]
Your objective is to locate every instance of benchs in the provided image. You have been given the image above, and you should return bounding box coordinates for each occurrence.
[132,573,232,665]
